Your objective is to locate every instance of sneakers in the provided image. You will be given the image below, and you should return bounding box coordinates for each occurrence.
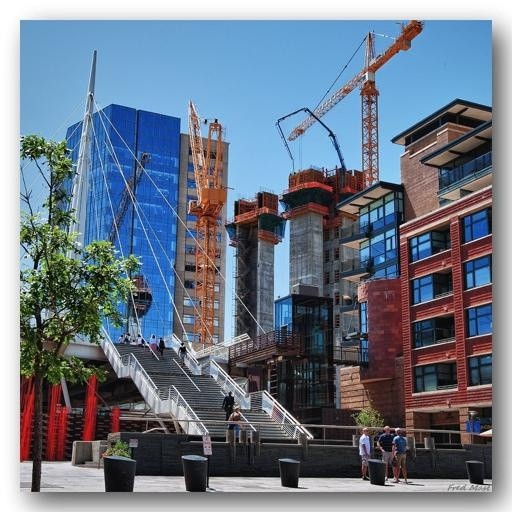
[363,476,369,480]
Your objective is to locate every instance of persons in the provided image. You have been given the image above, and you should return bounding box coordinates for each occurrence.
[118,333,142,345]
[178,343,187,366]
[377,426,407,484]
[150,335,165,356]
[222,391,244,442]
[359,427,371,480]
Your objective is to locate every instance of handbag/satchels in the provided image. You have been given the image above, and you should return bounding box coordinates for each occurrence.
[392,456,397,466]
